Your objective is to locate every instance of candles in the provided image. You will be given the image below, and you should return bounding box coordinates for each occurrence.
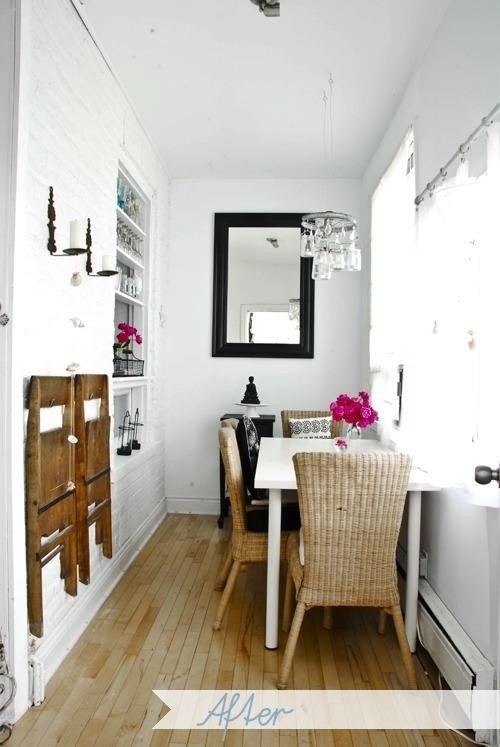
[101,253,116,270]
[70,217,87,249]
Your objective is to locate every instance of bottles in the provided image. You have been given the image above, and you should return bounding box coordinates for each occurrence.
[117,174,142,299]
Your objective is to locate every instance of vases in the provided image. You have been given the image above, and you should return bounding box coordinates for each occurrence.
[346,423,361,448]
[113,359,143,377]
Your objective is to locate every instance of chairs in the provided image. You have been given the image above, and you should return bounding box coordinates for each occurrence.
[213,426,295,631]
[219,420,268,571]
[280,409,343,439]
[276,451,420,692]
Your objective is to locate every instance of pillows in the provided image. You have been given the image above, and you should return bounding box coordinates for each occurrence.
[288,416,333,438]
[235,415,266,500]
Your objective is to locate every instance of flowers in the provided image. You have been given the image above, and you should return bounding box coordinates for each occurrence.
[330,391,379,428]
[114,322,143,356]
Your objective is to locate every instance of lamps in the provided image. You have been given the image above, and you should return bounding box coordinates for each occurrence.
[300,74,361,280]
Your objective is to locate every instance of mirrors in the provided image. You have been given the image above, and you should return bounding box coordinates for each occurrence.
[212,212,313,359]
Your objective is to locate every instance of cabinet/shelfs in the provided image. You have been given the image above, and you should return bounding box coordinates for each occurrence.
[117,160,155,459]
[217,414,275,528]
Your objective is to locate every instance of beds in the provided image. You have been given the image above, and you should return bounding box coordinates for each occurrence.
[253,437,435,653]
